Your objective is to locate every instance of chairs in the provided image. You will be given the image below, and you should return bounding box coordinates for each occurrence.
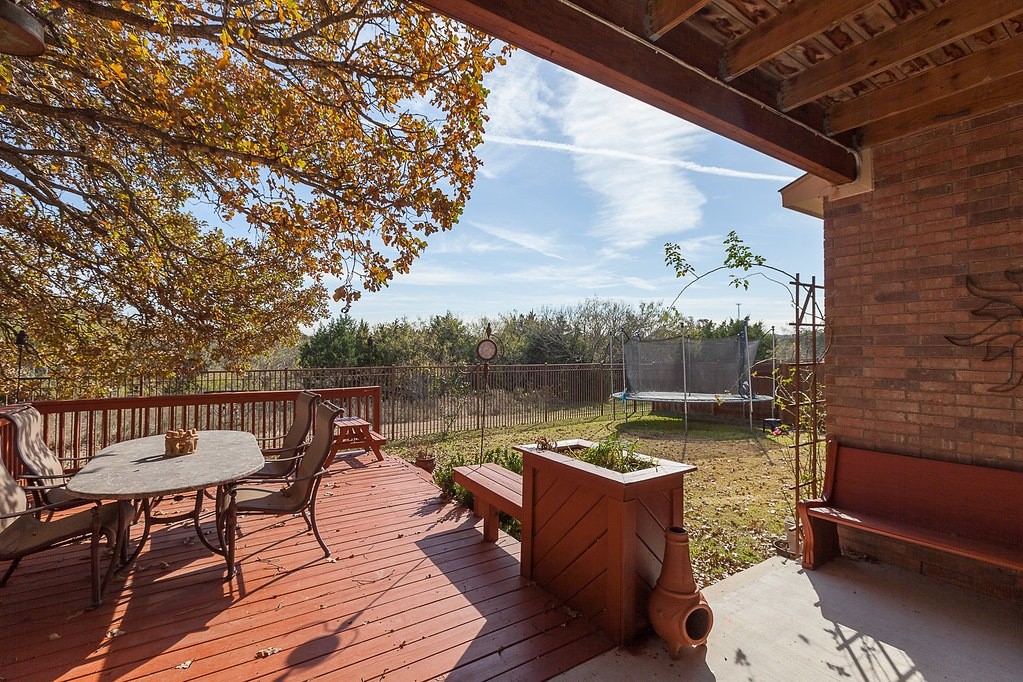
[0,403,133,559]
[215,400,345,582]
[216,389,322,530]
[1,452,136,608]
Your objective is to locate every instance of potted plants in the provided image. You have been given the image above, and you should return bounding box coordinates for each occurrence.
[510,420,698,648]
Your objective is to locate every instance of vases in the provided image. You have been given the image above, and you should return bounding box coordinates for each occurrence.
[416,456,436,473]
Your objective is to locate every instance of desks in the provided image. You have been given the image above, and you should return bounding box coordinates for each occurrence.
[323,416,384,471]
[67,430,265,611]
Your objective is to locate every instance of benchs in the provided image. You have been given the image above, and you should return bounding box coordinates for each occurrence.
[452,462,523,542]
[370,430,388,445]
[798,433,1023,575]
